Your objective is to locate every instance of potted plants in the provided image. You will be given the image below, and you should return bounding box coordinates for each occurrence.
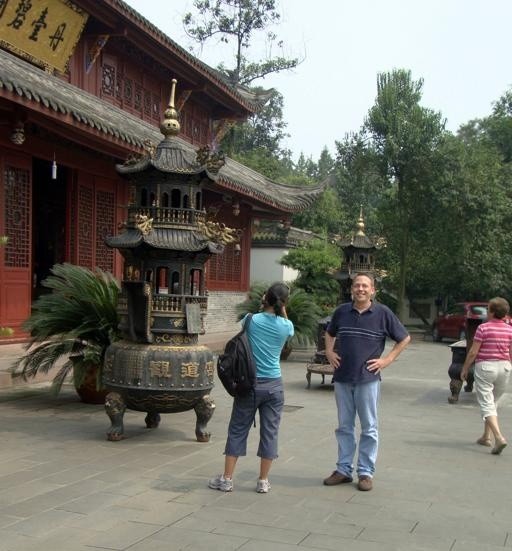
[6,262,121,405]
[234,281,323,360]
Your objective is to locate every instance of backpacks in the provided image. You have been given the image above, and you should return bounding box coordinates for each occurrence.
[216,312,257,397]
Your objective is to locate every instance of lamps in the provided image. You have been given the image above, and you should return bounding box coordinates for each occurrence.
[51,152,57,179]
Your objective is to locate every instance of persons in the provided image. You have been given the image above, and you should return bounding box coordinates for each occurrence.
[207,282,296,495]
[460,296,511,456]
[321,270,412,492]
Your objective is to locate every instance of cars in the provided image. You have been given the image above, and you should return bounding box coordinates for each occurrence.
[432,301,512,342]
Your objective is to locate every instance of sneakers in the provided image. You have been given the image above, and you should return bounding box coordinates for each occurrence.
[255,479,272,493]
[492,439,507,454]
[359,477,372,490]
[476,438,491,445]
[324,471,353,485]
[208,475,233,492]
